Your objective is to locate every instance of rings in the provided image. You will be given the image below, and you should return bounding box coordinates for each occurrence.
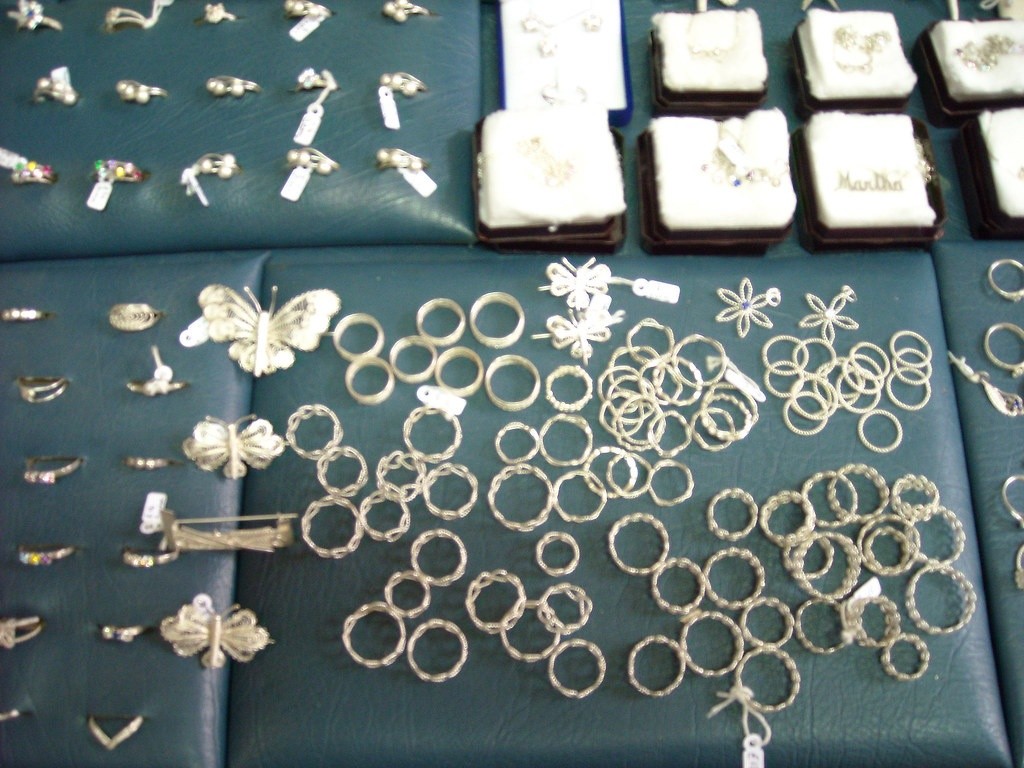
[0,0,426,188]
[1,252,1023,767]
